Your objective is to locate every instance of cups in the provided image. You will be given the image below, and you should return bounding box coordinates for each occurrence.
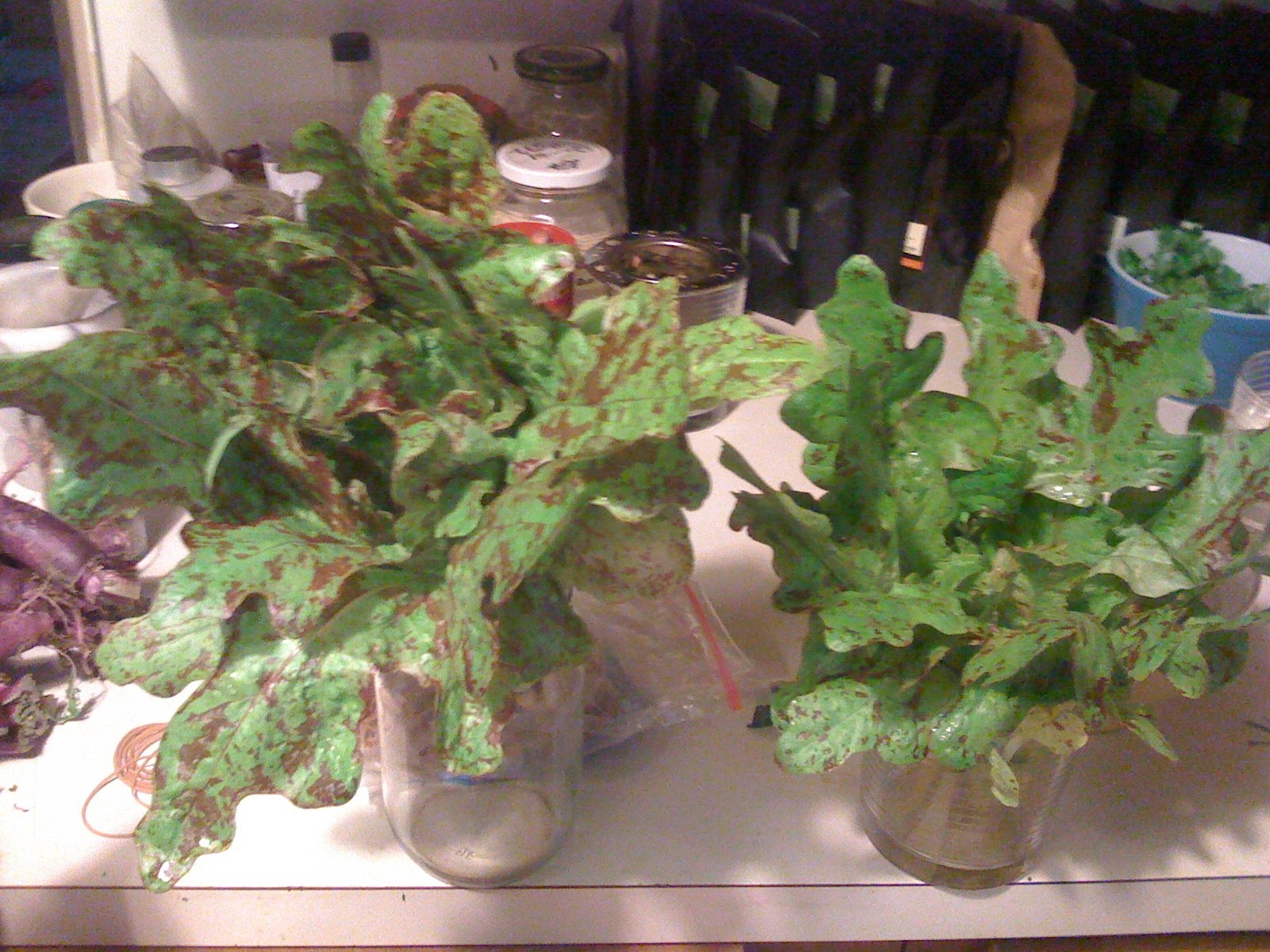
[1210,351,1270,530]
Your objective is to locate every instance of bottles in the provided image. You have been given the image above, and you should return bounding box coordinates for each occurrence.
[489,141,628,250]
[503,42,617,169]
[327,33,370,148]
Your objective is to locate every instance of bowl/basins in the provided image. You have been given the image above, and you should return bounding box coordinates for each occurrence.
[1109,229,1270,407]
[24,161,233,217]
[0,260,123,354]
